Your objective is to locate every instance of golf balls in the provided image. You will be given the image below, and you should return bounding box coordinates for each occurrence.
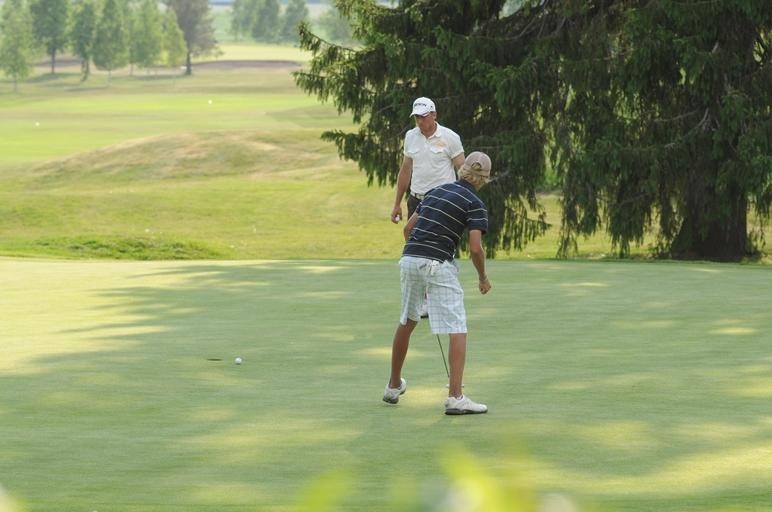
[235,358,241,365]
[395,217,399,222]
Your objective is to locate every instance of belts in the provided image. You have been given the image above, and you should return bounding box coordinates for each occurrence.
[410,191,424,200]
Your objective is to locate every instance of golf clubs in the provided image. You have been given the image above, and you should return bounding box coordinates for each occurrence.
[435,334,464,388]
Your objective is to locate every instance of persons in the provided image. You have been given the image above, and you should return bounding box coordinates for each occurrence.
[382,150,495,414]
[390,97,466,318]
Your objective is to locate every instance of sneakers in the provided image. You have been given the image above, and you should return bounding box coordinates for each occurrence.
[420,298,428,318]
[382,378,407,404]
[444,393,488,415]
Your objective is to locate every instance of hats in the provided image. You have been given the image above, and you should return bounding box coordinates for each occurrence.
[463,151,491,177]
[409,96,436,118]
